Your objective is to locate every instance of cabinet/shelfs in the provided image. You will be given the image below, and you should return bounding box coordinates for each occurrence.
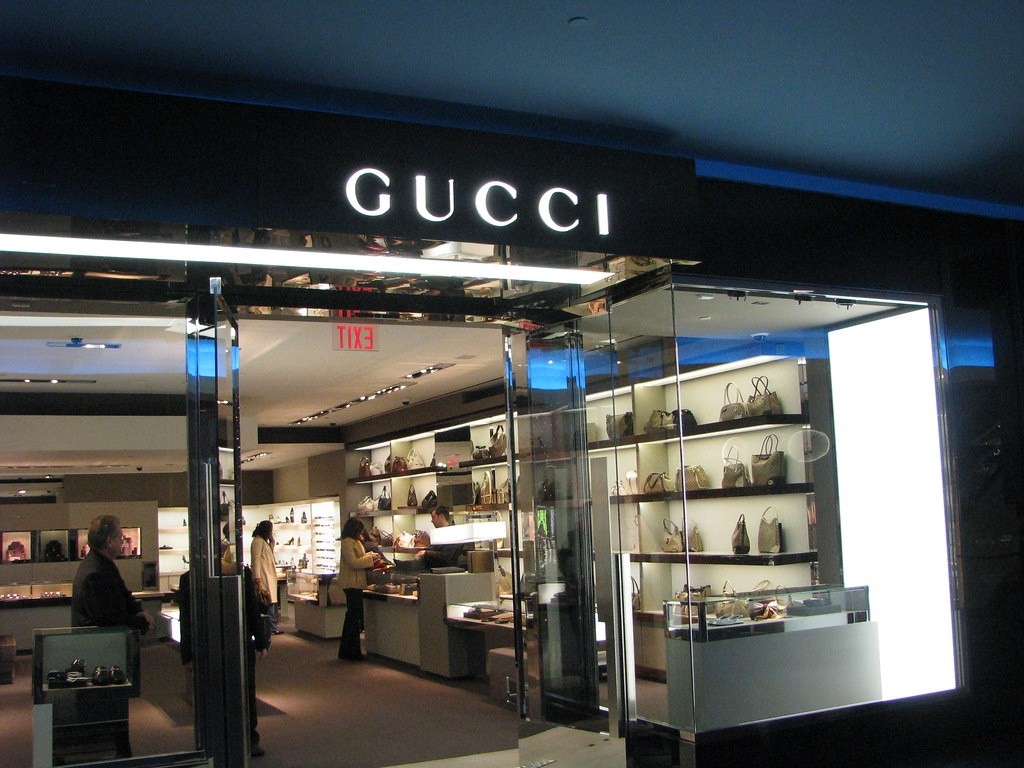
[157,494,339,576]
[355,415,582,558]
[586,409,824,565]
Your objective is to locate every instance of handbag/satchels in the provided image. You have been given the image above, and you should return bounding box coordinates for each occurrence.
[356,376,785,618]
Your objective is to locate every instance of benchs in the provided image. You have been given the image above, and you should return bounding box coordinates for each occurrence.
[490,646,536,705]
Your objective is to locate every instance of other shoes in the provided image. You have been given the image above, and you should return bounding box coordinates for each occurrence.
[252,743,264,756]
[272,630,285,634]
[159,505,310,570]
[348,654,367,661]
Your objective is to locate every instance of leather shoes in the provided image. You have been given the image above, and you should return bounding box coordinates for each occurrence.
[91,666,112,686]
[109,665,127,684]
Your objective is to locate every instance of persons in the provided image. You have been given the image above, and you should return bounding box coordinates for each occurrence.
[220,521,236,575]
[251,521,284,634]
[338,517,380,663]
[245,567,271,758]
[416,505,461,571]
[359,529,391,572]
[72,516,156,698]
